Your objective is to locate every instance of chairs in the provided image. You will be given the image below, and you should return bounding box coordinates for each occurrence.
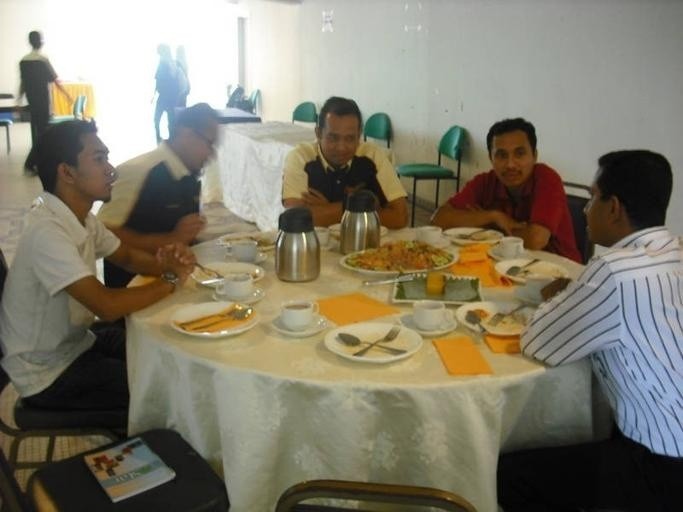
[249,89,260,113]
[0,112,14,154]
[398,124,464,228]
[275,479,478,512]
[0,427,231,512]
[47,95,86,124]
[292,101,319,126]
[364,112,392,149]
[563,181,595,265]
[0,249,130,475]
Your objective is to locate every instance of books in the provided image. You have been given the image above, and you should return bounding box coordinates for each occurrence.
[83,437,176,502]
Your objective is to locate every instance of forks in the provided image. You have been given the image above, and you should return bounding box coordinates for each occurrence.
[354,325,400,356]
[178,305,239,325]
[488,302,529,325]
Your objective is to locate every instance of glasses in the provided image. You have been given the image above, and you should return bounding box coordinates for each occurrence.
[196,131,220,153]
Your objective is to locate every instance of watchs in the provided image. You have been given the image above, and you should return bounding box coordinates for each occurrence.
[158,270,180,294]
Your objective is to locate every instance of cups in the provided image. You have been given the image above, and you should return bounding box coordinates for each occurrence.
[268,313,327,338]
[499,238,524,259]
[280,300,319,331]
[412,299,444,331]
[417,226,441,246]
[215,274,253,299]
[313,226,328,247]
[224,241,257,263]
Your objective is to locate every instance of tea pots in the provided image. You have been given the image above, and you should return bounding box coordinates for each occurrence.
[274,207,319,283]
[340,191,381,256]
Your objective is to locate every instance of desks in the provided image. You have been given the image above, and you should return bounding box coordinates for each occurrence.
[173,107,261,123]
[214,120,403,232]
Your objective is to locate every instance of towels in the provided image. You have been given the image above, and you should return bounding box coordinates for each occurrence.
[178,305,255,335]
[453,240,512,288]
[431,335,494,376]
[316,293,401,327]
[483,333,521,352]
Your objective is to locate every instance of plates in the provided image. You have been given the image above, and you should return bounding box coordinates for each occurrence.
[190,262,265,289]
[396,309,457,337]
[323,322,422,364]
[443,228,504,246]
[210,287,265,305]
[170,301,258,338]
[340,244,459,275]
[494,259,569,284]
[219,232,276,252]
[329,223,388,239]
[391,274,481,306]
[236,252,267,266]
[487,246,531,263]
[456,300,537,335]
[316,239,337,254]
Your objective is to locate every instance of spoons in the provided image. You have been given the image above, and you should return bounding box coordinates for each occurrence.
[456,230,490,238]
[466,309,489,336]
[506,259,539,275]
[363,274,415,287]
[191,306,252,329]
[336,332,407,356]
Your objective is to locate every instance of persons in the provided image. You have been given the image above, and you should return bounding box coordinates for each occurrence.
[497,147,683,511]
[174,45,189,108]
[281,94,410,230]
[430,116,584,268]
[152,44,180,143]
[13,30,75,176]
[96,103,221,329]
[1,115,200,411]
